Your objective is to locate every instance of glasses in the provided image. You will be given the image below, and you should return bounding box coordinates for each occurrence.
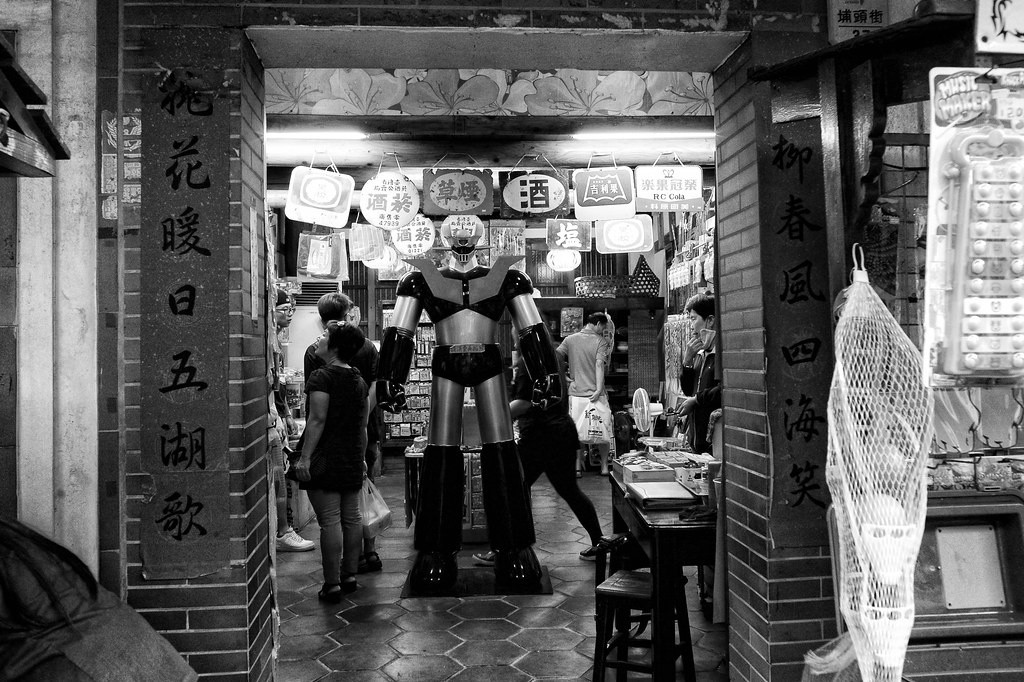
[337,320,347,331]
[275,308,296,316]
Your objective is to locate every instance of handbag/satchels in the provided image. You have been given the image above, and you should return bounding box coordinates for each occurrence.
[285,447,329,476]
[576,400,611,444]
[360,476,391,537]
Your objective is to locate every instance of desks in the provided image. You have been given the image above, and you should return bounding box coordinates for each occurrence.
[607,463,731,682]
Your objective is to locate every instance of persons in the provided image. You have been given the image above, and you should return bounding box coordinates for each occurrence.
[288,293,383,604]
[275,288,315,551]
[473,321,610,566]
[556,312,613,478]
[676,294,720,455]
[376,215,563,584]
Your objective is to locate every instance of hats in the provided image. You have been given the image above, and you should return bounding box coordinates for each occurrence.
[276,289,290,306]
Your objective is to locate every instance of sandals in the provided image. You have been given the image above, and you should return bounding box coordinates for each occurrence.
[601,468,613,476]
[575,469,583,478]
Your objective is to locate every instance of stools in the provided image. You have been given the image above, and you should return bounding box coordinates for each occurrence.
[592,566,698,682]
[592,527,655,638]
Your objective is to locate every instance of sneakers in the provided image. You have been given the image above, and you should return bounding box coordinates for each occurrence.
[274,528,315,553]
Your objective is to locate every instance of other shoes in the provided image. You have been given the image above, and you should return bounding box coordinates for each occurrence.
[580,548,610,561]
[342,574,360,593]
[472,553,495,564]
[318,583,342,604]
[358,551,383,573]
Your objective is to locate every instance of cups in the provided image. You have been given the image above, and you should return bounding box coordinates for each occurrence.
[549,321,556,330]
[713,478,721,509]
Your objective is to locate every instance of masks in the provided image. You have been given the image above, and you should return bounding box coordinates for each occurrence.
[700,320,717,349]
